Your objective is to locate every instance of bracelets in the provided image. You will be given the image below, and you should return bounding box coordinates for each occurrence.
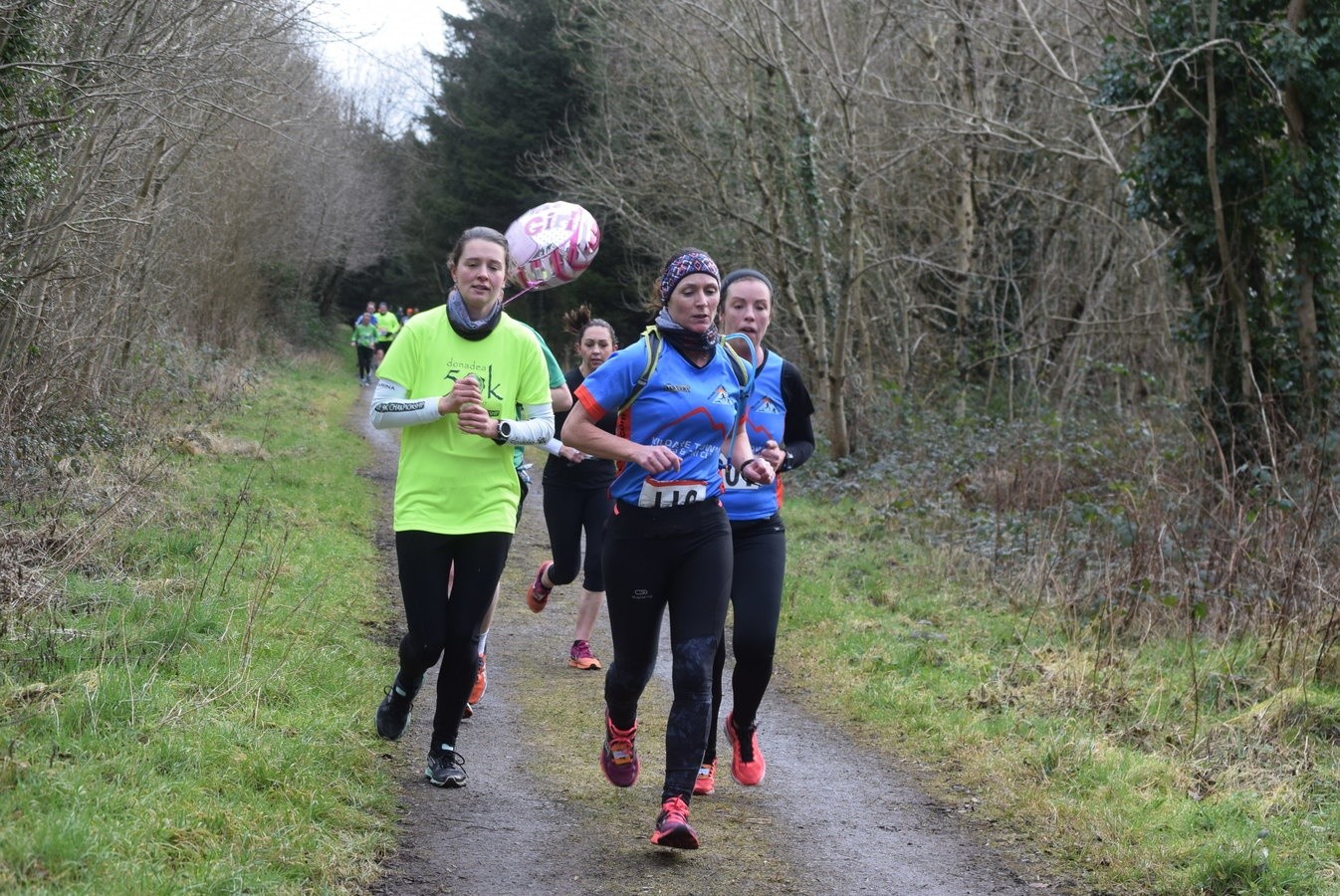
[739,458,764,488]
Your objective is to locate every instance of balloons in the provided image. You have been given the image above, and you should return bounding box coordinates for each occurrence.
[500,199,602,294]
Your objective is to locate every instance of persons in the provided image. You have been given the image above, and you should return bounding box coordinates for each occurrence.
[400,308,415,325]
[369,303,401,368]
[350,313,378,388]
[413,308,420,314]
[395,306,406,321]
[560,246,776,851]
[354,300,381,377]
[372,224,557,792]
[441,313,576,720]
[388,304,395,312]
[524,303,632,672]
[691,265,818,798]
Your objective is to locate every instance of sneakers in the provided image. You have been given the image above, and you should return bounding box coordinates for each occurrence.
[526,560,554,612]
[652,797,699,850]
[374,674,424,741]
[568,639,602,670]
[722,711,766,788]
[693,756,718,795]
[460,644,488,718]
[601,706,640,788]
[424,737,468,787]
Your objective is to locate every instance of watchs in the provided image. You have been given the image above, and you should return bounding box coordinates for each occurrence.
[491,419,513,446]
[780,448,796,473]
